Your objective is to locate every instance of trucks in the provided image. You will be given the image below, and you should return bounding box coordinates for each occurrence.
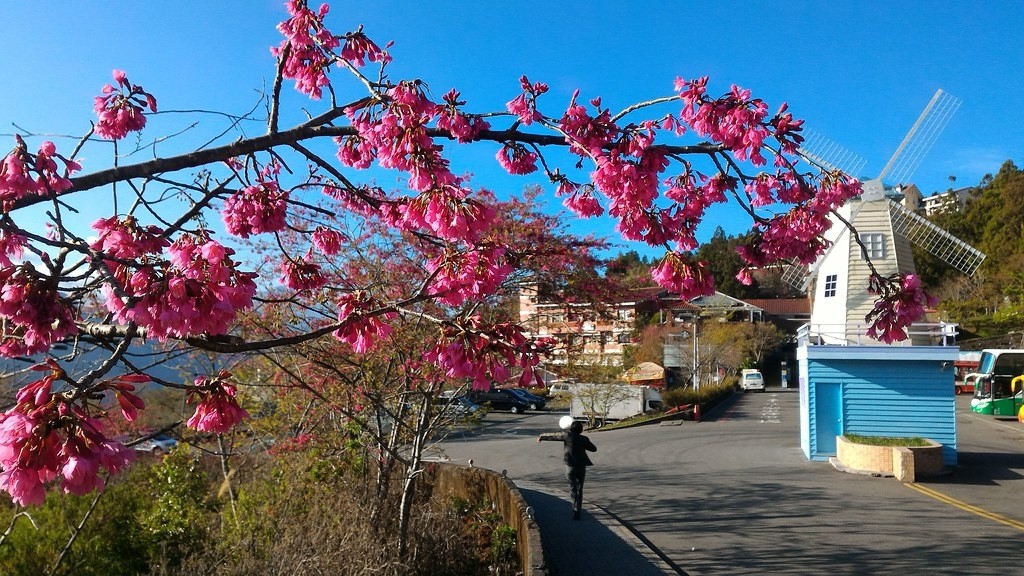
[569,384,665,430]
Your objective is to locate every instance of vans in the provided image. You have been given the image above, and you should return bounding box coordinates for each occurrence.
[744,372,765,393]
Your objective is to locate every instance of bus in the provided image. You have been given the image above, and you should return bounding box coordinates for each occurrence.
[1010,371,1024,424]
[955,351,983,396]
[964,349,1023,416]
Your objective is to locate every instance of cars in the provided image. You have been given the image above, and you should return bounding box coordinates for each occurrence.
[510,387,546,410]
[339,401,416,443]
[469,389,532,412]
[426,393,488,419]
[134,432,178,456]
[549,383,572,396]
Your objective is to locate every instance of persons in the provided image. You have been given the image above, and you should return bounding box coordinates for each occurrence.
[536,419,597,520]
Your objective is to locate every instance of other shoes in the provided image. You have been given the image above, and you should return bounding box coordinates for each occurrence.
[573,512,581,520]
